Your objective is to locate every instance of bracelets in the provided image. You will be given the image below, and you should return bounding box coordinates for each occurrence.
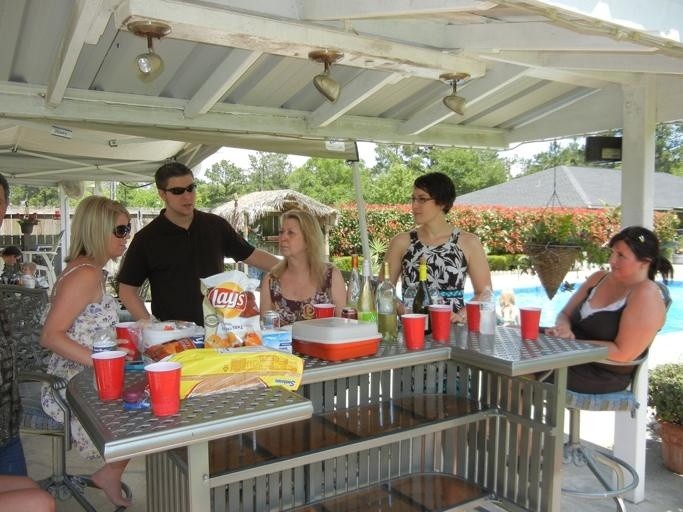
[456,311,466,321]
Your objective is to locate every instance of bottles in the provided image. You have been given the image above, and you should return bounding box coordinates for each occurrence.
[346,254,361,309]
[358,260,375,323]
[91,322,118,390]
[428,283,440,304]
[404,283,418,313]
[413,256,433,335]
[375,262,398,345]
[479,284,496,336]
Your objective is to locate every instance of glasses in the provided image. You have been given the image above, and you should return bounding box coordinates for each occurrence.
[113,223,131,238]
[160,183,196,194]
[408,196,435,204]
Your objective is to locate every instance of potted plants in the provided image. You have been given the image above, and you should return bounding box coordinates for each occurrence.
[646,362,683,474]
[520,216,609,296]
[18,219,38,234]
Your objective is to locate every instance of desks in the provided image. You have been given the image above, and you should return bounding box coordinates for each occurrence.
[62,314,610,512]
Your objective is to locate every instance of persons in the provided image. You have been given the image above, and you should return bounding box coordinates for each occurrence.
[0,477,54,512]
[496,226,672,472]
[0,174,27,476]
[375,170,495,398]
[0,244,21,286]
[116,162,284,327]
[258,208,349,403]
[38,195,132,508]
[11,261,39,290]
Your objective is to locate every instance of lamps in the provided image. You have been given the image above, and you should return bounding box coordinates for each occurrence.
[441,70,472,117]
[310,50,342,103]
[126,20,170,85]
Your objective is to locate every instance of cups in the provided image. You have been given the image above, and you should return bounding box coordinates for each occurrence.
[520,307,542,341]
[400,313,426,350]
[465,301,480,333]
[115,322,140,359]
[91,350,128,400]
[428,303,452,341]
[144,362,181,416]
[314,304,336,318]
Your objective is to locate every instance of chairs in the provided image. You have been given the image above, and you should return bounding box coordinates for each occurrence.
[0,281,131,512]
[541,281,673,512]
